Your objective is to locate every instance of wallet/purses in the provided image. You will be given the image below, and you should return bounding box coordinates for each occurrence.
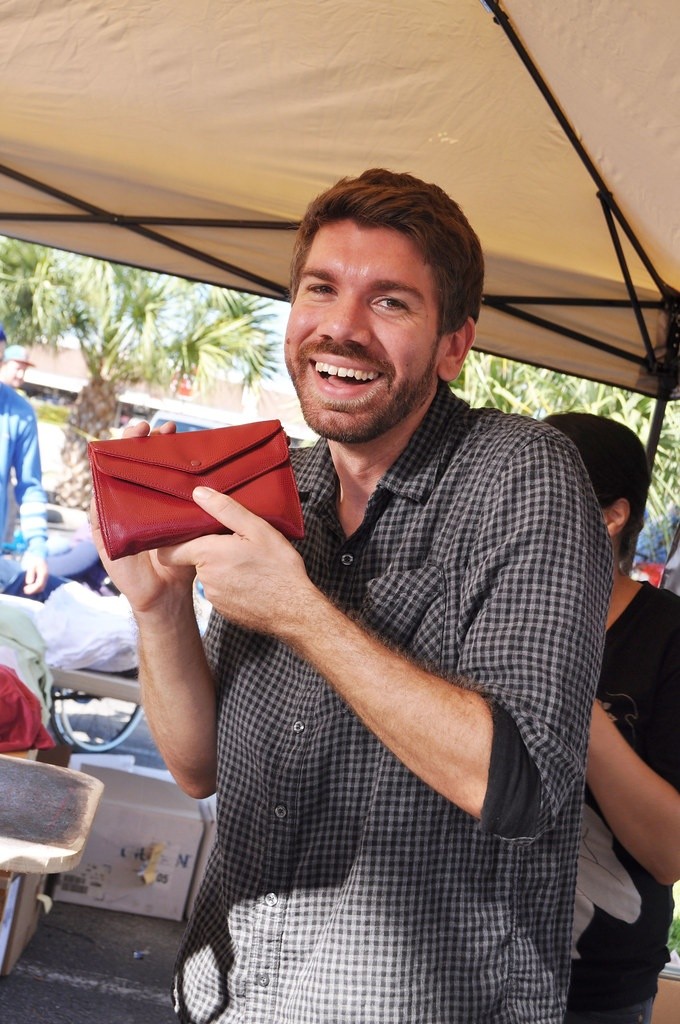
[86,418,306,562]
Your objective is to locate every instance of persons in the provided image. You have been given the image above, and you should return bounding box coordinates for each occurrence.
[93,168,615,1024]
[0,328,50,595]
[541,410,680,1023]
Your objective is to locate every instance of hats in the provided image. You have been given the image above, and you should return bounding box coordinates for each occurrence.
[4,344,39,369]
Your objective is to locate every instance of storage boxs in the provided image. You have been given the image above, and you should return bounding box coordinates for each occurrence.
[0,749,219,978]
